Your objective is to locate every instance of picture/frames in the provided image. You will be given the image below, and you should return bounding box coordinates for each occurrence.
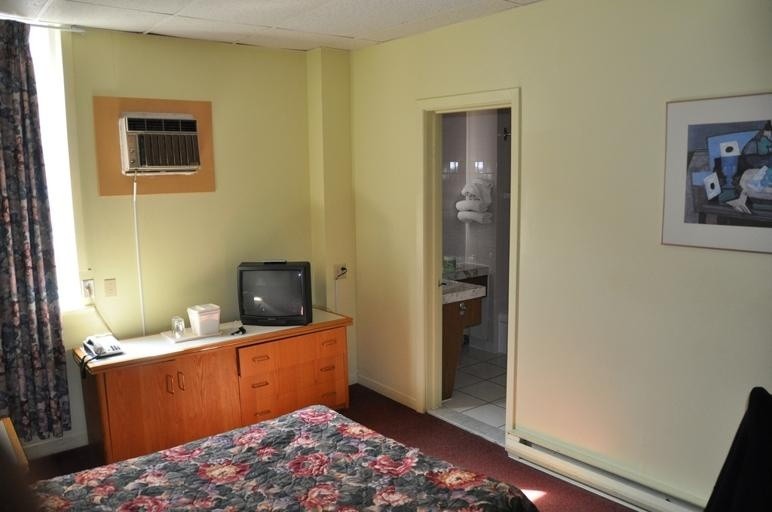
[661,92,772,255]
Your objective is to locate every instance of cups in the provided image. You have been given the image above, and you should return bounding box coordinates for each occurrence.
[172,315,185,340]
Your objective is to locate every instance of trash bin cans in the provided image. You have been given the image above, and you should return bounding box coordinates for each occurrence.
[460,335,469,363]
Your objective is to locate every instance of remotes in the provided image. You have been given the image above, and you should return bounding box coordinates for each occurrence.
[265,259,288,264]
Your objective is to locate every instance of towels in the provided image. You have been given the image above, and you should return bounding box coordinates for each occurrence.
[454,178,494,224]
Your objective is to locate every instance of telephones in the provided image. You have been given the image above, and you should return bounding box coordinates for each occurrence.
[83,333,122,360]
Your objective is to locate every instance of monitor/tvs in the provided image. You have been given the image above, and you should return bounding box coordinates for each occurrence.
[236,260,313,326]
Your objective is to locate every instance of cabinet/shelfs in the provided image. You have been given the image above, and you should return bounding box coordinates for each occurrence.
[72,304,352,467]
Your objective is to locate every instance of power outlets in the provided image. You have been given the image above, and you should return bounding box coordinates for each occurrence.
[334,263,347,280]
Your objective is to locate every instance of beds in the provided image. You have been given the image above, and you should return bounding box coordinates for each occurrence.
[26,404,536,512]
